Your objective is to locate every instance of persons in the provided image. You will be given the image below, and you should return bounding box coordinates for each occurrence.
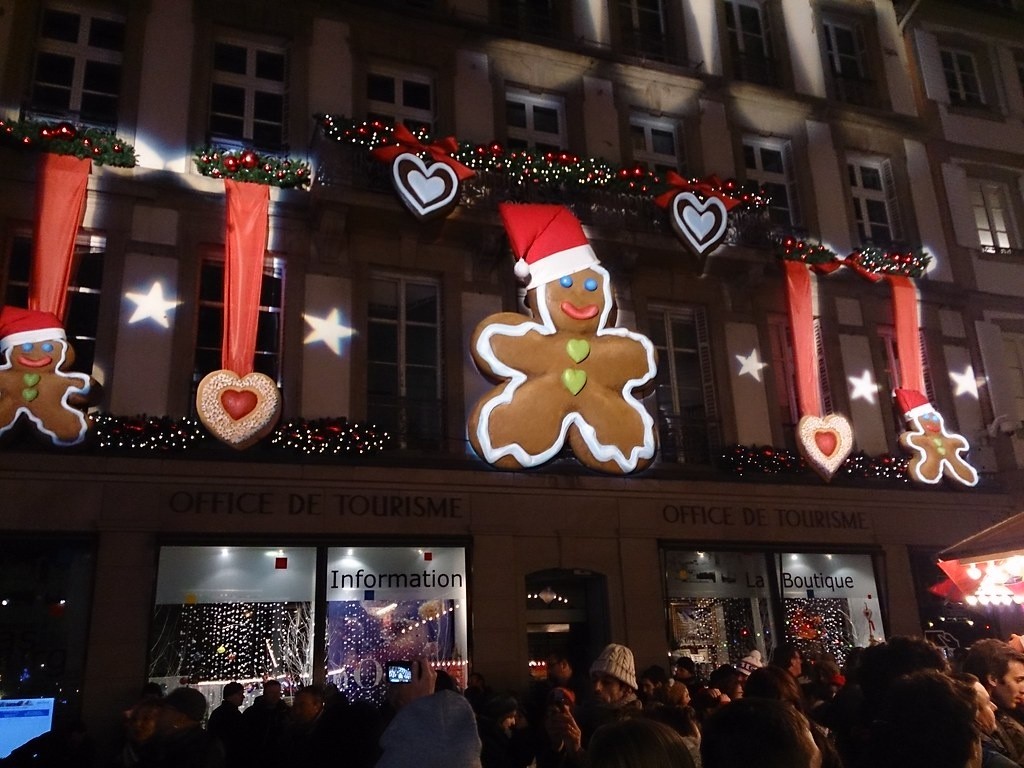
[0,634,1024,768]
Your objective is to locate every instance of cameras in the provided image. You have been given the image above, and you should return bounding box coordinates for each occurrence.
[384,660,421,684]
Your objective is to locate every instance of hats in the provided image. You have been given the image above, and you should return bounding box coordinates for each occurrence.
[153,687,205,722]
[549,686,575,706]
[675,658,696,675]
[736,649,763,676]
[590,644,637,691]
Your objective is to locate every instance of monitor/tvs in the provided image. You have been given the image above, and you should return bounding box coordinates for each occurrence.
[0,695,56,761]
